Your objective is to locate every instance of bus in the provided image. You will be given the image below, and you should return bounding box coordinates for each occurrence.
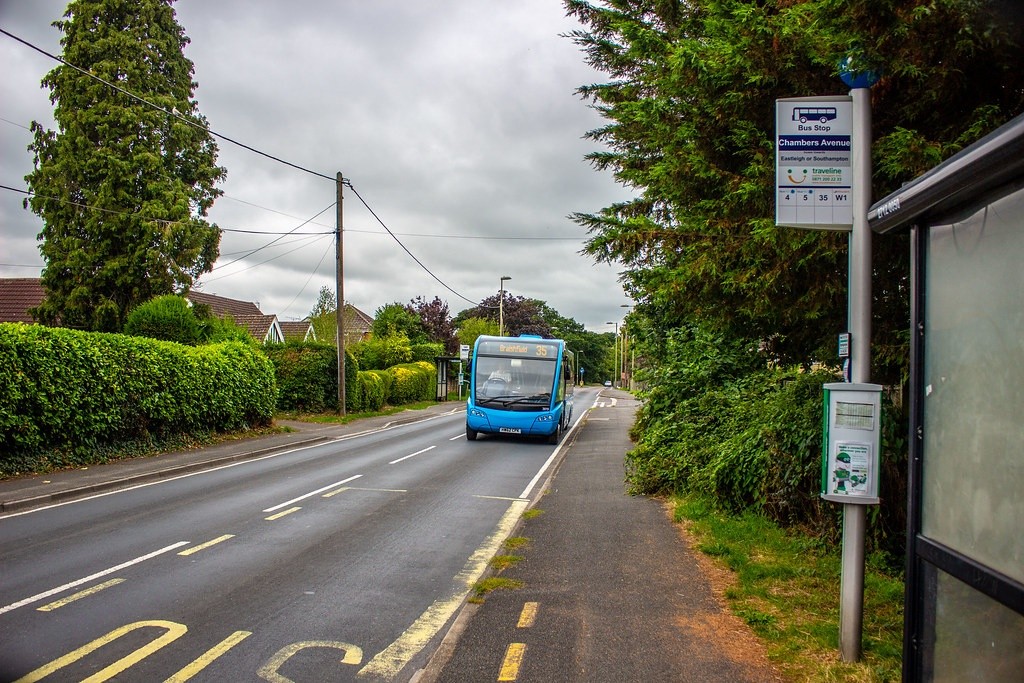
[466,334,575,448]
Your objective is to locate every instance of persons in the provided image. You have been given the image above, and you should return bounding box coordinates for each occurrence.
[489,360,511,385]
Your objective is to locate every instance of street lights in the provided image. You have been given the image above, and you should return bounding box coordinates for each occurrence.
[620,302,636,392]
[606,321,618,389]
[500,276,513,336]
[576,350,584,387]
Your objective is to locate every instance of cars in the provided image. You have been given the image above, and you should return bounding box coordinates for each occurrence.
[604,380,612,387]
